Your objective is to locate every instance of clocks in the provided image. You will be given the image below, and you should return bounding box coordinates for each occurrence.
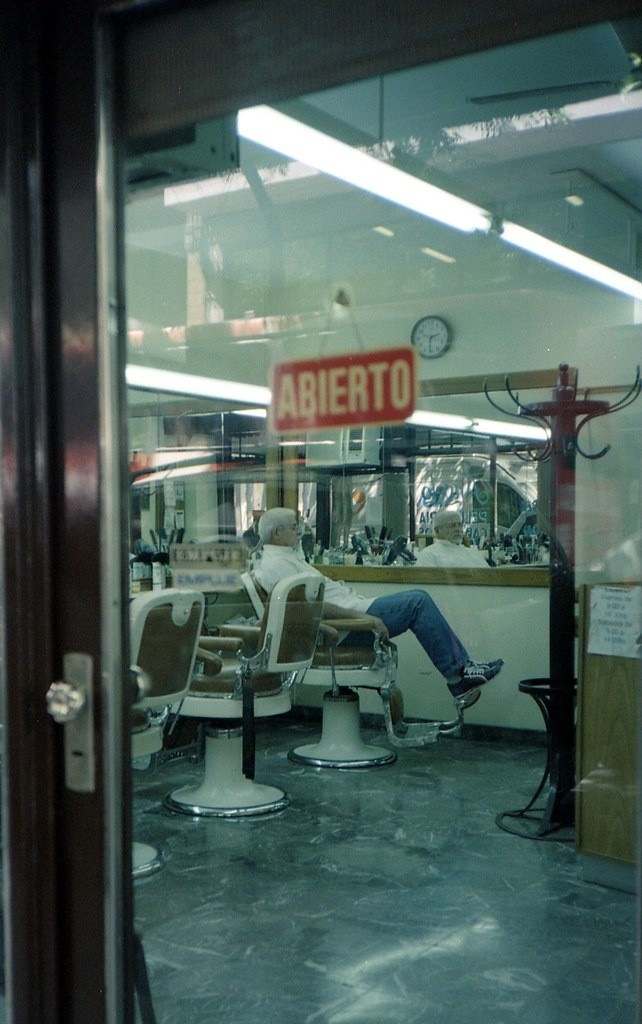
[409,315,452,360]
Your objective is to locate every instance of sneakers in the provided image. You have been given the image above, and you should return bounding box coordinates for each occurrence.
[447,659,505,700]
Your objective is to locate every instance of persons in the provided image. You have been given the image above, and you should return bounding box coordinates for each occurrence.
[242,519,264,551]
[256,508,504,697]
[297,510,314,563]
[416,510,492,568]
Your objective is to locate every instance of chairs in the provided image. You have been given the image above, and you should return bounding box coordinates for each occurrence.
[163,570,339,818]
[128,586,223,879]
[239,569,484,773]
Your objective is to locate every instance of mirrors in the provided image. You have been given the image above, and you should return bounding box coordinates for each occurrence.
[124,363,579,590]
